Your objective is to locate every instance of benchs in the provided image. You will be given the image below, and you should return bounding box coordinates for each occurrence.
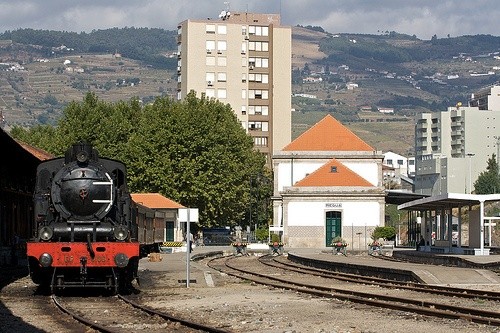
[463,248,490,256]
[418,246,449,254]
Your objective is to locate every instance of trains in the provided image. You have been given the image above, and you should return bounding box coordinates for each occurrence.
[27,142,164,291]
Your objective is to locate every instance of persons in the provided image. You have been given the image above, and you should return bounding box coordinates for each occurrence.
[184,232,193,254]
[416,236,425,251]
